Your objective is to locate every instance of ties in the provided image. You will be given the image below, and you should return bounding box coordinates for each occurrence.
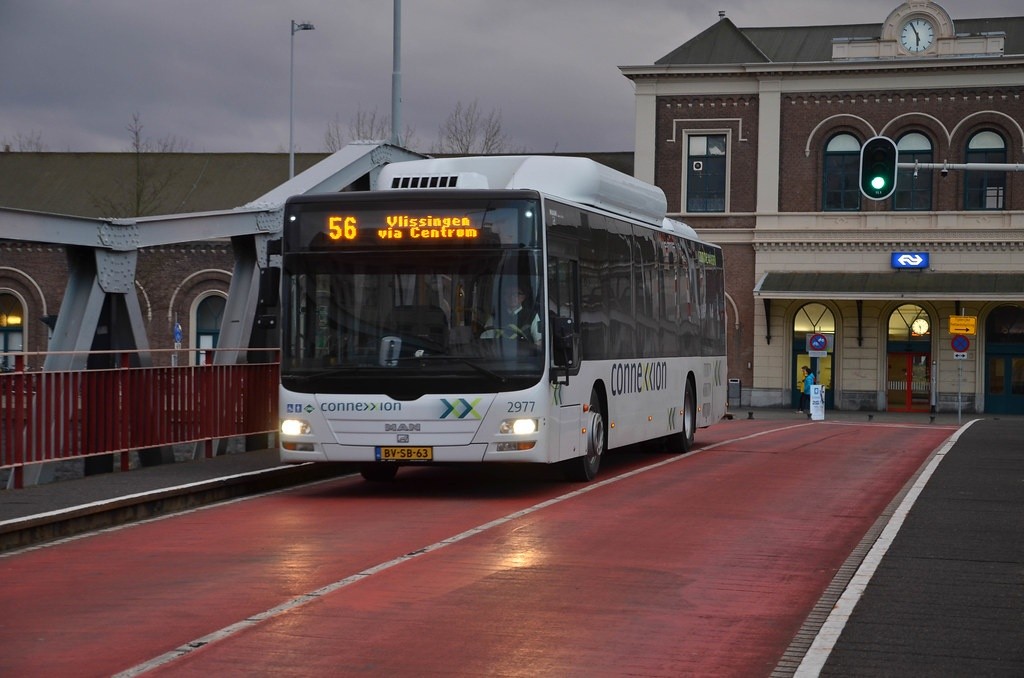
[511,311,514,318]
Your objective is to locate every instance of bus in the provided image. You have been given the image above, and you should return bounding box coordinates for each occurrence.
[269,155,728,482]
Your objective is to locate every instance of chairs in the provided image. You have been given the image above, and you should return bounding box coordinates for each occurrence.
[383,305,450,355]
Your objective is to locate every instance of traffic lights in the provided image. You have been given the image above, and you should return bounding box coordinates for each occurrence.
[861,136,898,200]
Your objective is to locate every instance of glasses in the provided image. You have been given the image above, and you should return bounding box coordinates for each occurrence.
[505,292,524,297]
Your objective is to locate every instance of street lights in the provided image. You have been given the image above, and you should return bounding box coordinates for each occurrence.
[290,21,315,197]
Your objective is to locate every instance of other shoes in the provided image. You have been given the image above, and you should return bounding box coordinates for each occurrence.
[794,410,803,414]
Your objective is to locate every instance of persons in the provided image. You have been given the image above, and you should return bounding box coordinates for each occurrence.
[483,285,543,346]
[793,365,815,413]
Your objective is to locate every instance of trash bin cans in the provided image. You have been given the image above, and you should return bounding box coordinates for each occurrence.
[728,378,740,406]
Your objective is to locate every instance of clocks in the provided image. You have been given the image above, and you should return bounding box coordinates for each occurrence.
[901,20,933,50]
